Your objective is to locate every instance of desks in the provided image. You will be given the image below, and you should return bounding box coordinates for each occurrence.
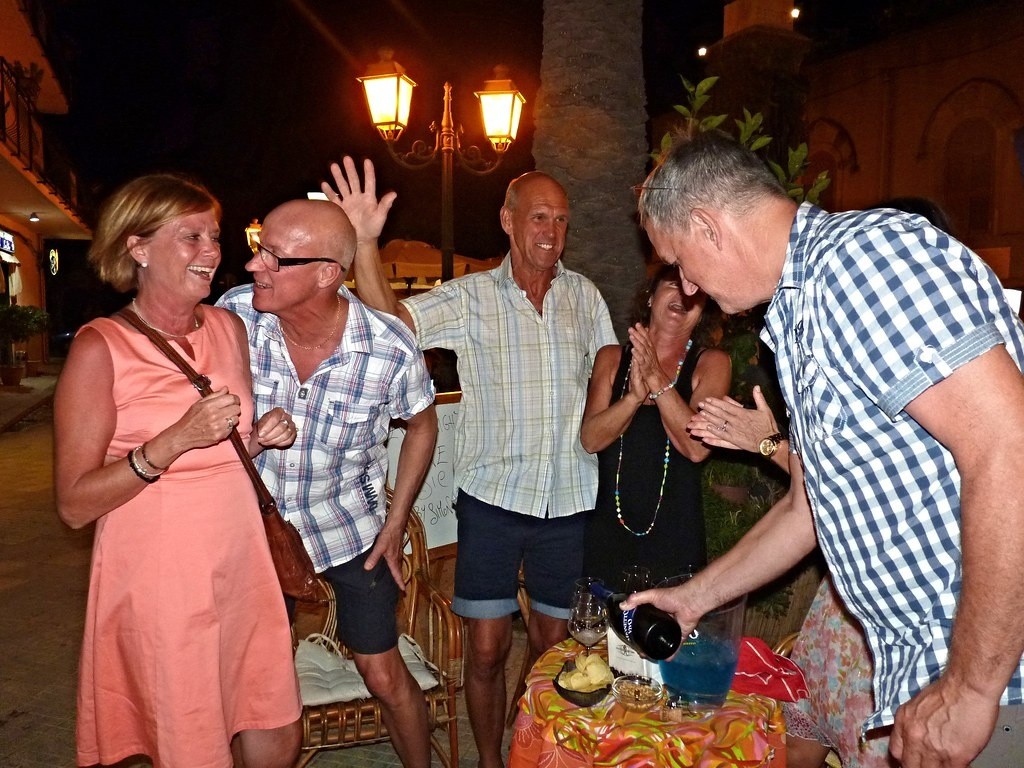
[507,627,787,768]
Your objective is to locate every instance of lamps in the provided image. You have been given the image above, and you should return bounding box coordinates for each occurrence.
[29,212,40,222]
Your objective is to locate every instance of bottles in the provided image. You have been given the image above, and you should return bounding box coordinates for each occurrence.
[586,581,682,660]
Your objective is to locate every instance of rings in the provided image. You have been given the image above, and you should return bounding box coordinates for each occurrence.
[280,406,289,418]
[226,418,233,429]
[723,421,728,432]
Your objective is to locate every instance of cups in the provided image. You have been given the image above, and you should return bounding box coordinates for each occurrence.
[657,574,747,709]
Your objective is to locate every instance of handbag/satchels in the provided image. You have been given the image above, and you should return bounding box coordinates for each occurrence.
[261,499,318,602]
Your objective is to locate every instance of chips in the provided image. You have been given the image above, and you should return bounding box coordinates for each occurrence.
[557,653,615,692]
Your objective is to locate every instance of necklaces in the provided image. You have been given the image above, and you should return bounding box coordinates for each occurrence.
[133,299,198,338]
[615,339,692,536]
[281,294,340,350]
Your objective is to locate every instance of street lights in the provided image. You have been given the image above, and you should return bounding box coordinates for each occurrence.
[354,46,527,283]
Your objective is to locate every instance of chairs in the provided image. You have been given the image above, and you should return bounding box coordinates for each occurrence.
[289,485,463,768]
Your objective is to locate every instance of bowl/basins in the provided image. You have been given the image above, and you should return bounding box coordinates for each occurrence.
[611,674,666,713]
[552,658,610,709]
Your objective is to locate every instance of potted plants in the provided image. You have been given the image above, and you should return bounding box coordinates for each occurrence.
[0,303,50,385]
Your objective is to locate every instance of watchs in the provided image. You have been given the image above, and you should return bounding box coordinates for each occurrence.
[759,433,786,457]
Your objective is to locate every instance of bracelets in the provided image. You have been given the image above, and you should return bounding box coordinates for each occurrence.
[126,441,169,484]
[649,384,674,400]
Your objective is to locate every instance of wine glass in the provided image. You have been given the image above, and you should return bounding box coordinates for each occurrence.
[569,566,652,659]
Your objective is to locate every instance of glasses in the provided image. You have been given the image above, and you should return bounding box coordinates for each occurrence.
[632,182,686,197]
[254,243,347,274]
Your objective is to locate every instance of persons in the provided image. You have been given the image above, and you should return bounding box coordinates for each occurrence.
[620,136,1024,768]
[580,263,733,595]
[54,175,304,768]
[214,198,432,768]
[687,198,947,768]
[321,155,616,768]
